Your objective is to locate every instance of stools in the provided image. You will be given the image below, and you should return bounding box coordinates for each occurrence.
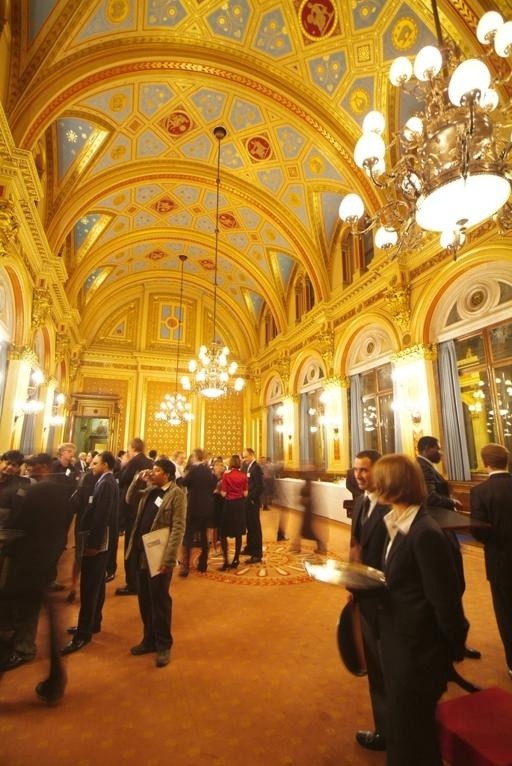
[437,687,512,764]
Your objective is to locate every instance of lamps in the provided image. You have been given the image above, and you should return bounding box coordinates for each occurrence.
[337,0,512,260]
[153,255,195,429]
[179,127,245,402]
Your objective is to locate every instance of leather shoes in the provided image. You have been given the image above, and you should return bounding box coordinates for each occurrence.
[3,627,170,701]
[356,730,385,751]
[106,551,262,595]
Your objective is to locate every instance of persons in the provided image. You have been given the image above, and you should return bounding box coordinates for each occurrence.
[358,452,471,765]
[171,448,276,575]
[1,438,189,699]
[290,466,327,556]
[471,442,511,675]
[415,434,483,659]
[347,452,394,751]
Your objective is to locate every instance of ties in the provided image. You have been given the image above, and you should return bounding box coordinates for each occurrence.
[360,496,371,527]
